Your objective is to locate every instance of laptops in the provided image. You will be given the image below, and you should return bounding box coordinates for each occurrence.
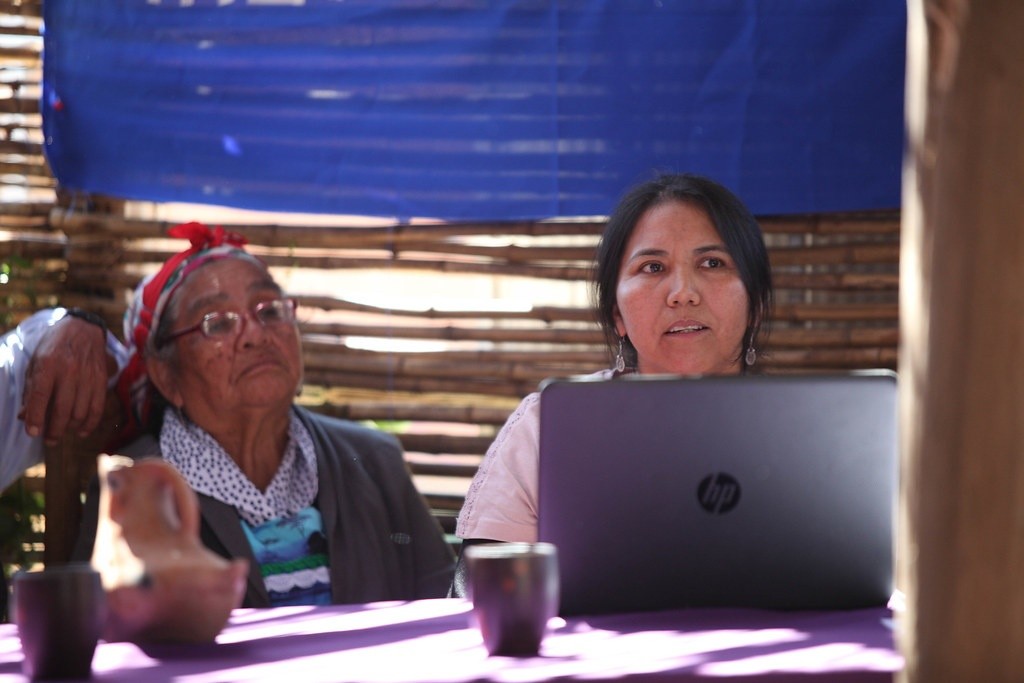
[535,367,901,620]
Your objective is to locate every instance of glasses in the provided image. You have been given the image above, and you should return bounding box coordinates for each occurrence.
[154,297,298,354]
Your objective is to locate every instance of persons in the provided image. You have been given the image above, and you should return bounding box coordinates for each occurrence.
[0,221,130,624]
[453,172,777,616]
[81,239,455,608]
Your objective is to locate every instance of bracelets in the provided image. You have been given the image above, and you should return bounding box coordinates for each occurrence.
[58,304,109,344]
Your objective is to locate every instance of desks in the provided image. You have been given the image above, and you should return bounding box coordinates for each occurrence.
[0,598,903,683]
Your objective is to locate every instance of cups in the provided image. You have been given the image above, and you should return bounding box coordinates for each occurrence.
[10,569,103,682]
[464,542,563,657]
